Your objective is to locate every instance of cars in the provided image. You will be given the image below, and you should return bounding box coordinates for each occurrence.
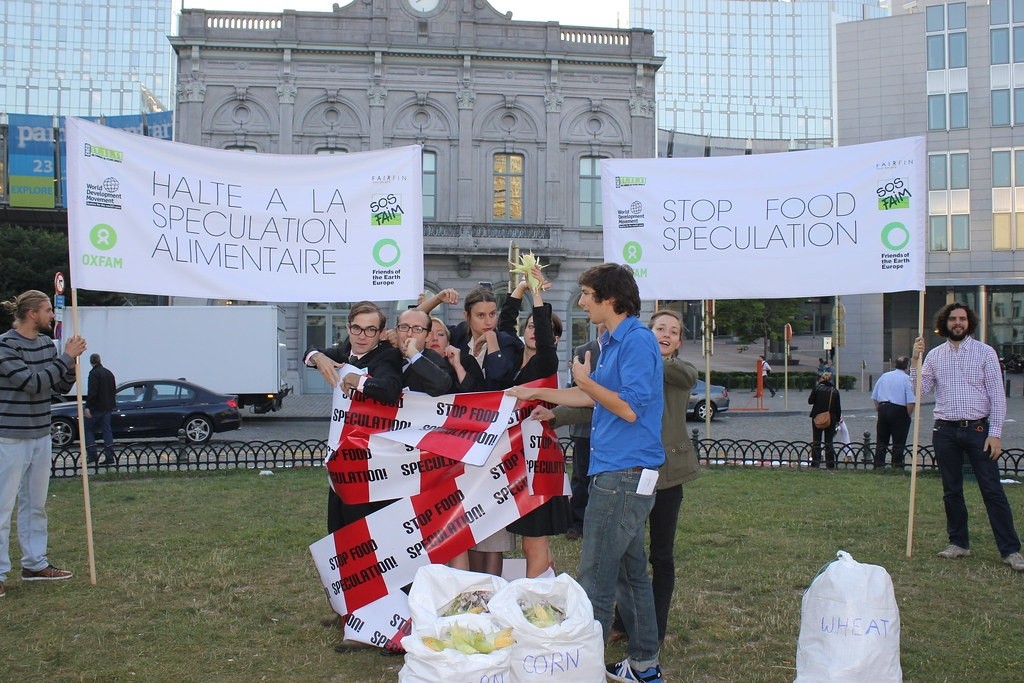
[48,378,242,450]
[686,379,731,422]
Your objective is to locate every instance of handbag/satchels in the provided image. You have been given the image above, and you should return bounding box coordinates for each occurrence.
[813,412,830,428]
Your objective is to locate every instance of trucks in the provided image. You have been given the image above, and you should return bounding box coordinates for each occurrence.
[47,305,295,417]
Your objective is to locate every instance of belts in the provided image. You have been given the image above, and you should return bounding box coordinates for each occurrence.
[936,419,987,427]
[880,402,894,407]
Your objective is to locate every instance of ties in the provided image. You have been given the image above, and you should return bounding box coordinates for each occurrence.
[402,360,409,367]
[349,356,358,364]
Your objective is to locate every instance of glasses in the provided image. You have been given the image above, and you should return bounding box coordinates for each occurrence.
[395,324,429,334]
[349,324,382,338]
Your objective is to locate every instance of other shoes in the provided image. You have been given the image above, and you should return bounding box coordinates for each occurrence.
[607,628,627,646]
[753,396,760,399]
[81,459,97,464]
[98,461,114,465]
[937,544,971,558]
[568,524,583,540]
[1002,552,1024,569]
[770,392,776,398]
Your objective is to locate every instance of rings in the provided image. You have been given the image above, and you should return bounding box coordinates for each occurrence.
[919,346,920,348]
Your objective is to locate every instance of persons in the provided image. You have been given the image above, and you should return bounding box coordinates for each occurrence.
[504,262,665,683]
[0,289,87,598]
[871,356,915,471]
[134,386,143,402]
[300,263,564,657]
[816,358,835,384]
[753,355,776,397]
[529,311,699,641]
[567,324,608,538]
[84,353,117,464]
[910,302,1024,570]
[807,372,842,469]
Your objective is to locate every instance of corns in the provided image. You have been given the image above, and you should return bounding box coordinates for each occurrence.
[420,604,553,654]
[522,253,538,293]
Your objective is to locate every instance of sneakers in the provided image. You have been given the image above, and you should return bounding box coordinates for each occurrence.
[604,661,662,683]
[22,565,73,580]
[0,581,7,596]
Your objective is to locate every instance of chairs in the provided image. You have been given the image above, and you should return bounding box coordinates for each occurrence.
[152,387,158,400]
